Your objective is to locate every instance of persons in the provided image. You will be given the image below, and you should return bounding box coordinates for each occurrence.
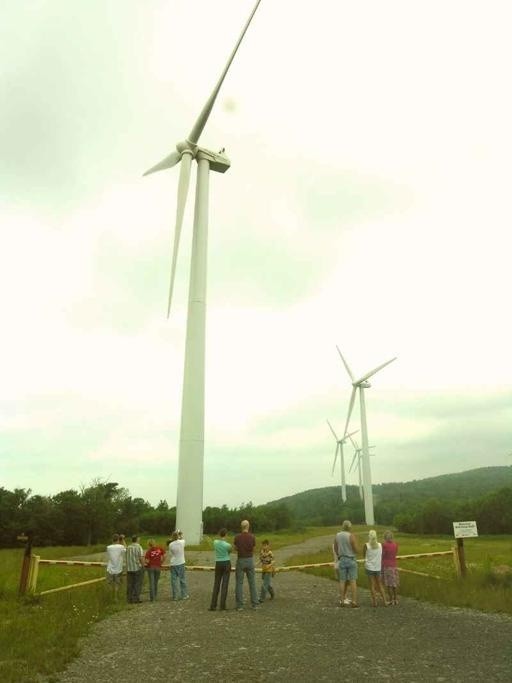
[144,539,167,601]
[331,531,353,605]
[126,535,146,602]
[232,518,263,612]
[380,528,399,606]
[257,539,278,603]
[333,518,361,608]
[363,529,389,607]
[107,532,128,602]
[168,528,191,601]
[208,528,234,612]
